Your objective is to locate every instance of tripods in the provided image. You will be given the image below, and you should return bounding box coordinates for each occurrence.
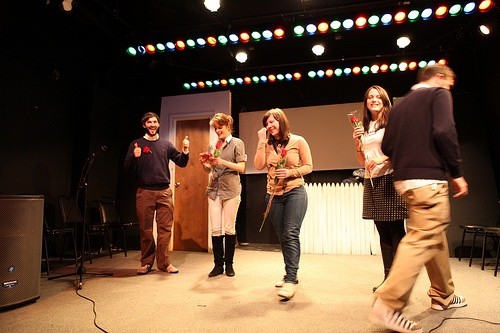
[47,155,113,290]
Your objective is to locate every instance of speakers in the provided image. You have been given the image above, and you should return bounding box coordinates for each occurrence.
[0,194,45,307]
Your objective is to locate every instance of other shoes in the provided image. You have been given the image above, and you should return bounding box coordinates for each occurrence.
[157,263,179,273]
[277,282,295,300]
[275,280,284,287]
[137,264,151,274]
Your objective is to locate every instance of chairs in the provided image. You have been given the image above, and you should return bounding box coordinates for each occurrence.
[458,224,500,277]
[43,195,139,275]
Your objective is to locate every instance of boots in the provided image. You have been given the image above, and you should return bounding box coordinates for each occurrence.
[225,233,236,276]
[208,235,225,277]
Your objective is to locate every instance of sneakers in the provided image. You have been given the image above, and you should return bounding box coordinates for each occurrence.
[431,294,467,311]
[367,297,424,333]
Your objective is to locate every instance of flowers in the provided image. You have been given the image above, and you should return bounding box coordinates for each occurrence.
[258,148,288,231]
[215,141,223,157]
[349,115,374,188]
[143,146,152,155]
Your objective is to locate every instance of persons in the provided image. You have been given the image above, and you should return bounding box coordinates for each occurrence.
[370,65,469,333]
[253,108,313,299]
[198,113,247,276]
[125,112,190,274]
[352,86,408,293]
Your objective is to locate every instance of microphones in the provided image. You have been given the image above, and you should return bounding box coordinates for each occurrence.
[91,145,107,157]
